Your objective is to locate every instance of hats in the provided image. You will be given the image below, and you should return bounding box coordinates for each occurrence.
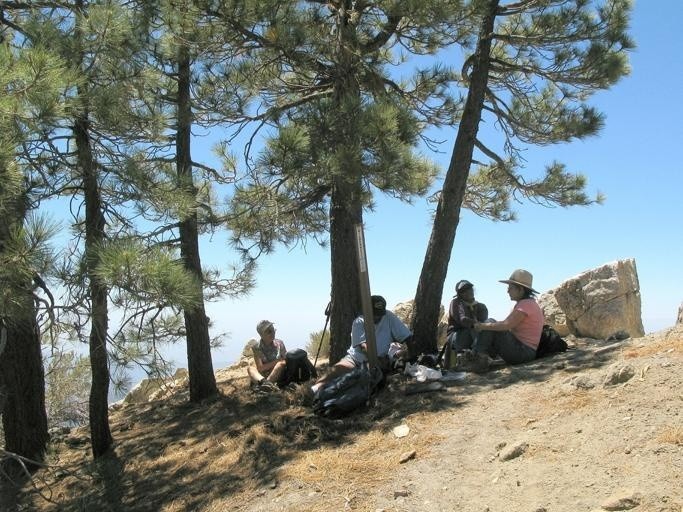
[497,268,540,294]
[371,295,386,317]
[256,319,274,336]
[453,280,473,298]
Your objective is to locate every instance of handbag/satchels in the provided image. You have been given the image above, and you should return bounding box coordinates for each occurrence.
[536,326,567,358]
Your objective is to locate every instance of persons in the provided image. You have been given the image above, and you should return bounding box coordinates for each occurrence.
[246,320,287,392]
[305,295,412,393]
[474,269,544,364]
[446,279,496,349]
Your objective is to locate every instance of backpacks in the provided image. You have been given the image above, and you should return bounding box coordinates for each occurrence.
[286,348,317,384]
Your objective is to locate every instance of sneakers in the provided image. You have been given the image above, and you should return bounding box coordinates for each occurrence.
[253,380,274,392]
